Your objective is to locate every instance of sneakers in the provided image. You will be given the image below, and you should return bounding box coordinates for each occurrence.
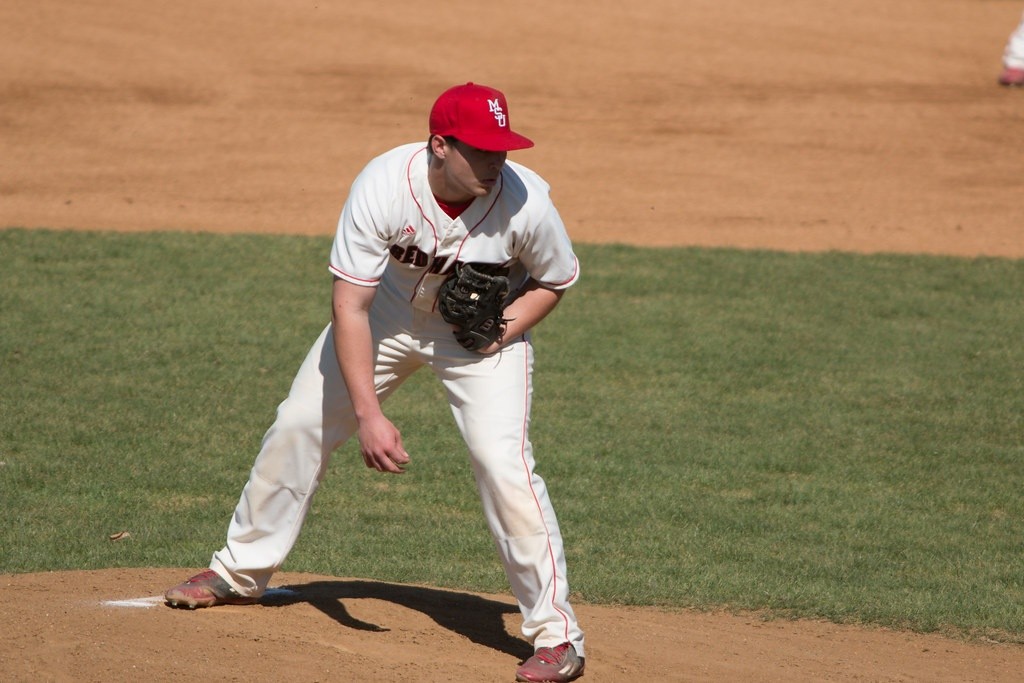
[164,570,258,609]
[514,643,585,683]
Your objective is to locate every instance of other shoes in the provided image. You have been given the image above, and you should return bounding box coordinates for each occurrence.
[1000,64,1023,86]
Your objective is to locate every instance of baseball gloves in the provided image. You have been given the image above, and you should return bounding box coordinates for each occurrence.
[437,260,512,352]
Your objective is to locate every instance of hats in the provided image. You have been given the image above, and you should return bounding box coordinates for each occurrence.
[429,82,535,152]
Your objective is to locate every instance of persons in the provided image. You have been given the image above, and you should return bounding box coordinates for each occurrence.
[164,82,585,683]
[997,21,1024,86]
